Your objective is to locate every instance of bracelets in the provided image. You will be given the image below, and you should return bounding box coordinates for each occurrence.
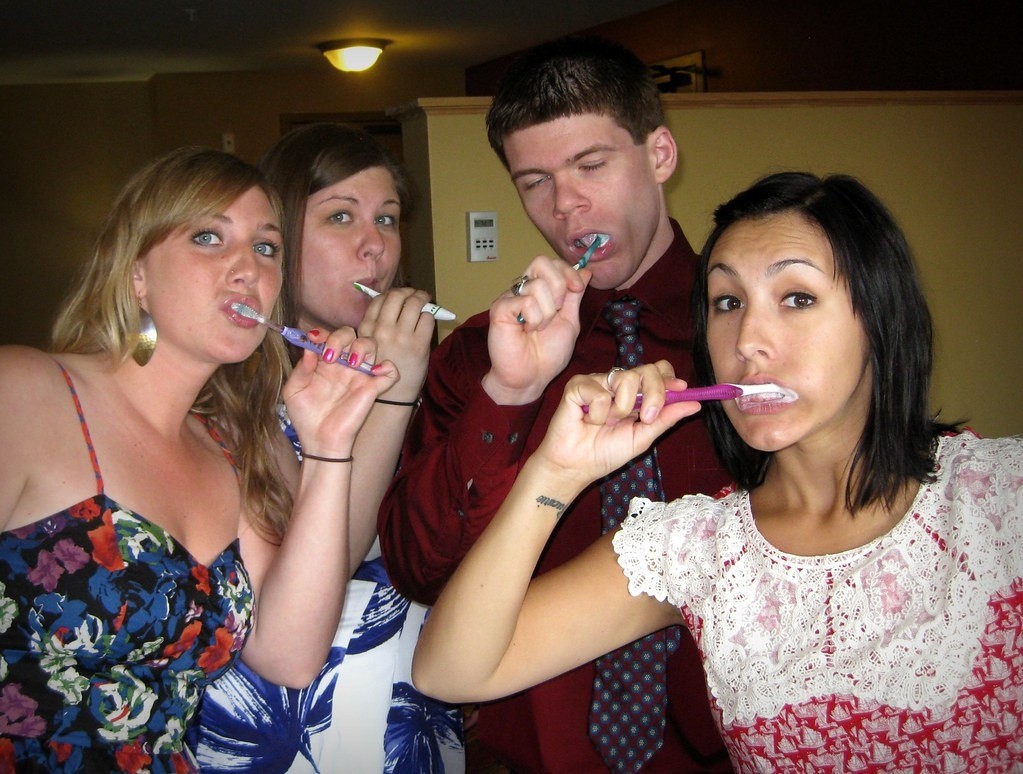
[375,398,419,406]
[299,452,353,462]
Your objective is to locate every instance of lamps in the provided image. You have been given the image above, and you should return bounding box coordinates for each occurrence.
[318,38,385,73]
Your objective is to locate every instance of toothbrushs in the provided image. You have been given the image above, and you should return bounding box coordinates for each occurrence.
[353,282,458,321]
[517,234,609,324]
[583,382,789,415]
[230,300,376,376]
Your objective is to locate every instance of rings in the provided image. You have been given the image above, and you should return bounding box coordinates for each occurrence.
[511,274,529,296]
[607,367,624,388]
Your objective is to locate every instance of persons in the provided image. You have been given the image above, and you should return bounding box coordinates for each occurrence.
[0,122,479,774]
[411,173,1023,774]
[378,34,730,774]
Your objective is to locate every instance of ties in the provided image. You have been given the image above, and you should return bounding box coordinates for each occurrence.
[589,292,680,773]
